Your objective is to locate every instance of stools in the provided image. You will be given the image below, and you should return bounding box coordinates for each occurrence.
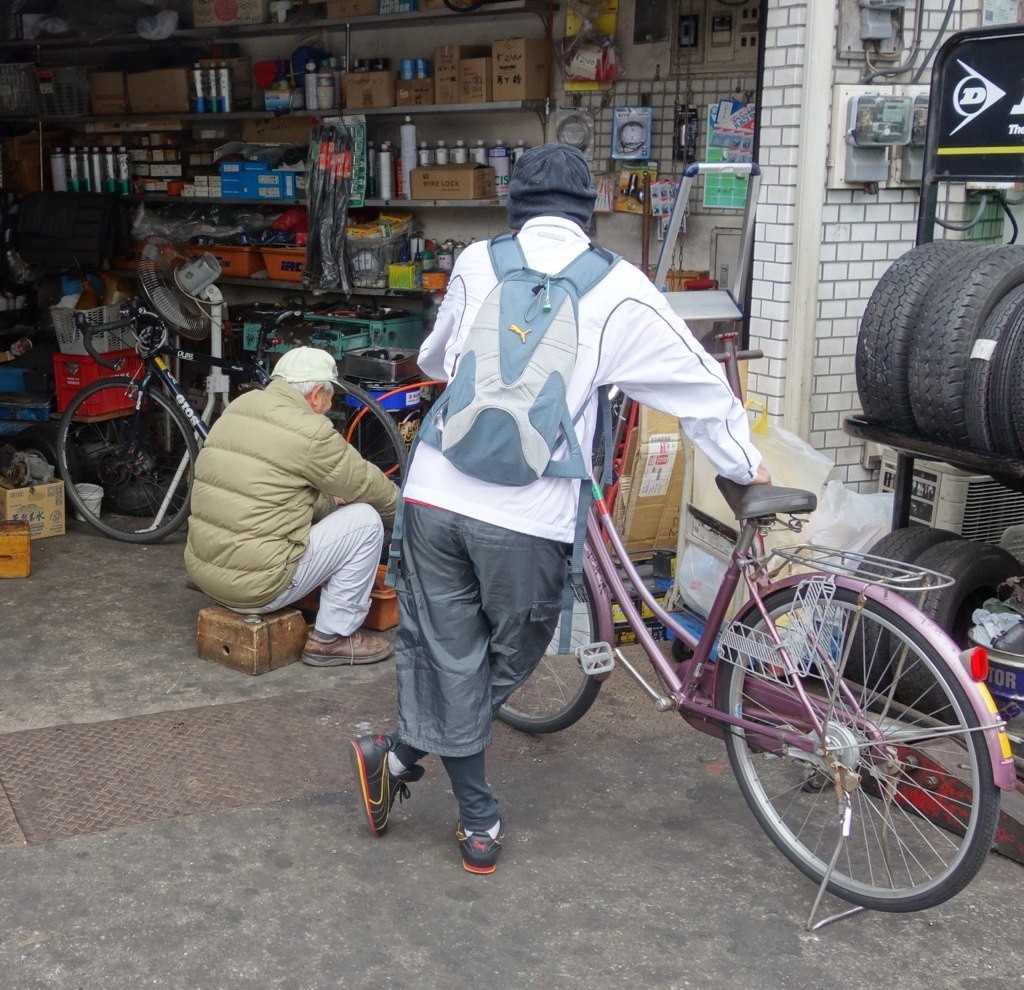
[197,605,308,676]
[0,520,31,579]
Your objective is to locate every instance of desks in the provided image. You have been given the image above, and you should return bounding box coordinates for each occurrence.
[51,406,151,462]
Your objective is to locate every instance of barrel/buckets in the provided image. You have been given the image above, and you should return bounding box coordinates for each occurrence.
[73,483,104,521]
[968,628,1023,755]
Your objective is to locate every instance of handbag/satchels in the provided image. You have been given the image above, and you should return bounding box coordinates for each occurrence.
[679,402,835,634]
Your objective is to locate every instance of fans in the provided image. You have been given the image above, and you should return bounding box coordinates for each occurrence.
[134,232,230,534]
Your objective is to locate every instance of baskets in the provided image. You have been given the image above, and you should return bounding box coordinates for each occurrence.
[48,301,138,355]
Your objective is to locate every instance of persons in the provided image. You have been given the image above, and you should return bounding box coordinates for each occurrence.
[348,141,775,874]
[185,346,402,666]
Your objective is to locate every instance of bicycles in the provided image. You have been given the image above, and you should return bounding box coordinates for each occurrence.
[496,380,1019,933]
[54,303,406,545]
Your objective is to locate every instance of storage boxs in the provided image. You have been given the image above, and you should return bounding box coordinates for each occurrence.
[0,0,551,288]
[293,564,398,630]
[0,474,66,538]
[0,301,145,421]
[345,348,422,412]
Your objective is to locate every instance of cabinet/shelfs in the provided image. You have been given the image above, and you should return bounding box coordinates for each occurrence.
[0,0,563,454]
[243,309,425,419]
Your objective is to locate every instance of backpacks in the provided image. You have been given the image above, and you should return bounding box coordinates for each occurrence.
[441,232,623,486]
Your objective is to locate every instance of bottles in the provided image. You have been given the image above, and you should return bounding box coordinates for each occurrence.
[189,61,233,114]
[410,230,476,272]
[304,54,367,111]
[50,144,131,195]
[367,114,527,201]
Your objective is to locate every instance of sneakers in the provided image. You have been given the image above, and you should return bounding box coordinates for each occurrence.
[350,734,425,837]
[456,810,504,873]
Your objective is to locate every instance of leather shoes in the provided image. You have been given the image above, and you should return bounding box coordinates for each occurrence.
[302,631,394,666]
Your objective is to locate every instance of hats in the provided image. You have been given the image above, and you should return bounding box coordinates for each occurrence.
[271,346,349,392]
[507,143,597,229]
